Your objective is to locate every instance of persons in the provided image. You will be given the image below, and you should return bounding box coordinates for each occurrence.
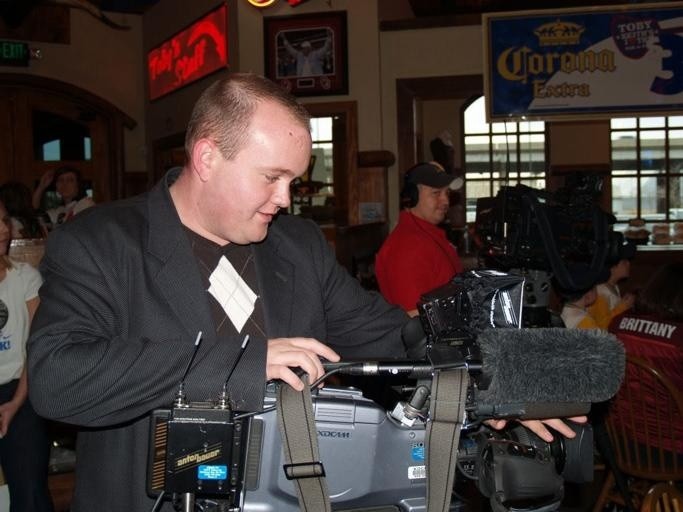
[280,33,332,77]
[585,250,635,330]
[0,196,57,511]
[554,262,601,331]
[22,71,589,511]
[428,128,463,206]
[374,160,464,320]
[32,163,96,227]
[604,259,682,479]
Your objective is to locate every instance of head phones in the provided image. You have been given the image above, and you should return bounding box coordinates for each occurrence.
[398,163,422,208]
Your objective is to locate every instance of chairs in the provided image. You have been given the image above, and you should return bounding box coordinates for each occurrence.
[590,355,682,512]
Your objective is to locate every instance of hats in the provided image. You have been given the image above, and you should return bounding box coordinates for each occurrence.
[549,261,612,302]
[403,161,465,191]
[606,230,638,263]
